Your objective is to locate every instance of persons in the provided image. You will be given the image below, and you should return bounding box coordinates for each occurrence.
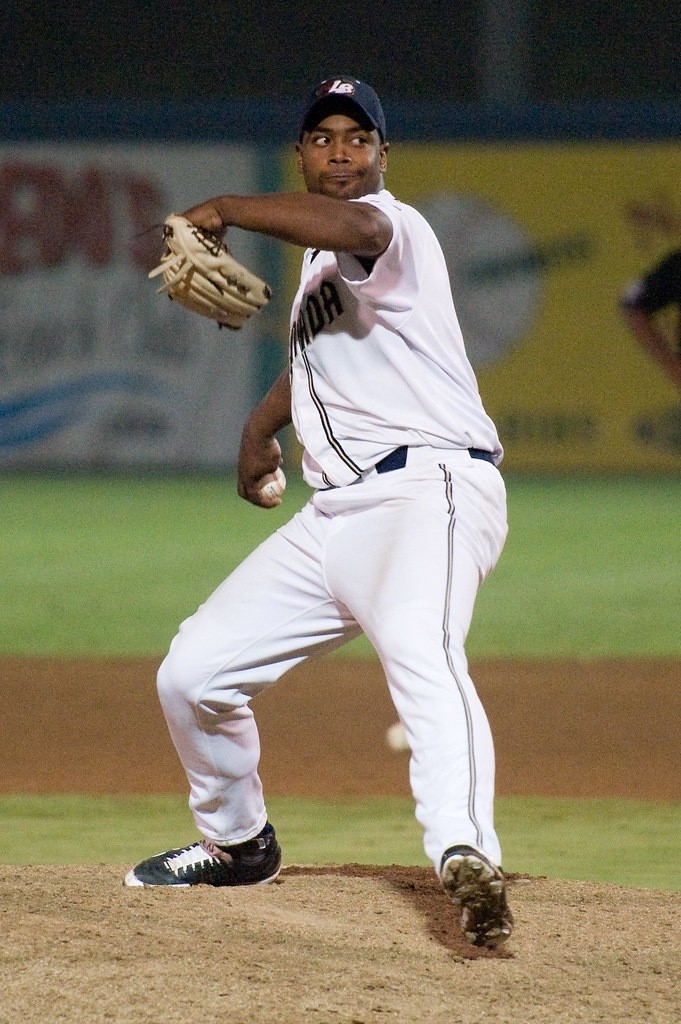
[617,246,681,392]
[121,75,515,950]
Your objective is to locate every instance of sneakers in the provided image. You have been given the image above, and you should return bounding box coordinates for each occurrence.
[436,843,516,947]
[124,822,284,889]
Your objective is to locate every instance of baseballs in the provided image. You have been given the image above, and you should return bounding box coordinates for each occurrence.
[258,466,288,502]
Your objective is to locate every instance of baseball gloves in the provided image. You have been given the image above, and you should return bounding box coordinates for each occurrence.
[154,213,272,331]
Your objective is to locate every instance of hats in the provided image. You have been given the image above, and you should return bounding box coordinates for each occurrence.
[300,73,388,143]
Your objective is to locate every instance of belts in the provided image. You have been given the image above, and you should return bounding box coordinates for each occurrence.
[374,444,495,473]
